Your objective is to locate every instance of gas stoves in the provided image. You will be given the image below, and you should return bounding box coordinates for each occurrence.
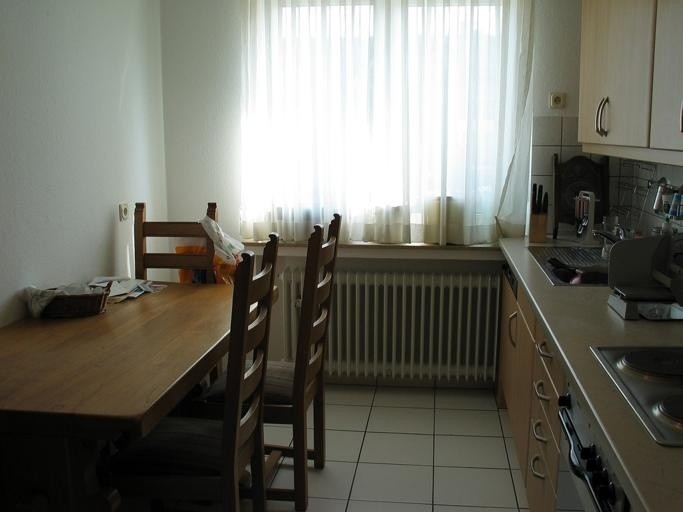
[589,341,683,449]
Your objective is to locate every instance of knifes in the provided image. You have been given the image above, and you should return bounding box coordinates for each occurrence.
[532,183,548,216]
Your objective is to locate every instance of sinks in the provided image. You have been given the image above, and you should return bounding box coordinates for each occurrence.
[546,261,609,288]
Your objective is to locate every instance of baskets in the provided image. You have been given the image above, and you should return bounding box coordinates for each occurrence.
[39,281,112,319]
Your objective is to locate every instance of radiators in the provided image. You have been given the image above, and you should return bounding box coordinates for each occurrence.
[276,265,501,386]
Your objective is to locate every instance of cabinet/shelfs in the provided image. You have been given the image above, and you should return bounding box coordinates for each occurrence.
[577,0,683,166]
[496,268,683,512]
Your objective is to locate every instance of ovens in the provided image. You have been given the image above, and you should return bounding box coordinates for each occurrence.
[555,372,643,511]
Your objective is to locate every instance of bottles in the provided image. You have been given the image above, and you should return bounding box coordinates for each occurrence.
[661,218,672,234]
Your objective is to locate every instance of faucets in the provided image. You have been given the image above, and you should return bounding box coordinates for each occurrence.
[590,229,621,245]
